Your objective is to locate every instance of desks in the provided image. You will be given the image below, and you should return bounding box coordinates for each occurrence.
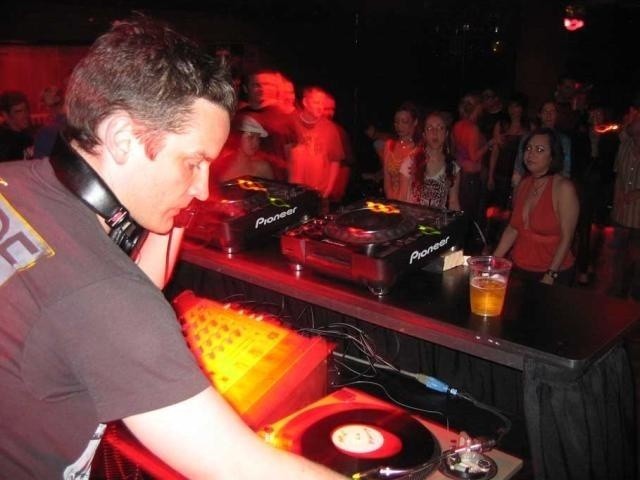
[179,218,640,479]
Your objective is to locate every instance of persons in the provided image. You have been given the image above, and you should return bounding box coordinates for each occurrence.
[486,95,537,203]
[359,112,389,142]
[469,87,510,208]
[571,105,619,287]
[609,118,640,300]
[231,73,248,114]
[551,74,594,184]
[321,94,350,215]
[0,16,356,478]
[208,114,274,192]
[493,126,581,286]
[379,105,420,205]
[508,99,572,198]
[290,86,342,216]
[0,93,40,161]
[398,111,463,211]
[234,69,295,158]
[450,94,508,214]
[619,103,640,141]
[277,81,301,121]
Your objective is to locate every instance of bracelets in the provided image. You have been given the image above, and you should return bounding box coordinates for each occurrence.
[544,270,562,281]
[485,142,495,151]
[319,193,327,201]
[22,149,32,160]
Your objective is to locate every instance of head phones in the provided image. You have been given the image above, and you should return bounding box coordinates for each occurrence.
[46,141,145,263]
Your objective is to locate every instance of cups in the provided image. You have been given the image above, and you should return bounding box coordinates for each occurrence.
[466,255,513,317]
[469,312,502,341]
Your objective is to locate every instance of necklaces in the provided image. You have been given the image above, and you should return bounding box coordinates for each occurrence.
[297,110,320,130]
[396,134,414,149]
[528,176,549,195]
